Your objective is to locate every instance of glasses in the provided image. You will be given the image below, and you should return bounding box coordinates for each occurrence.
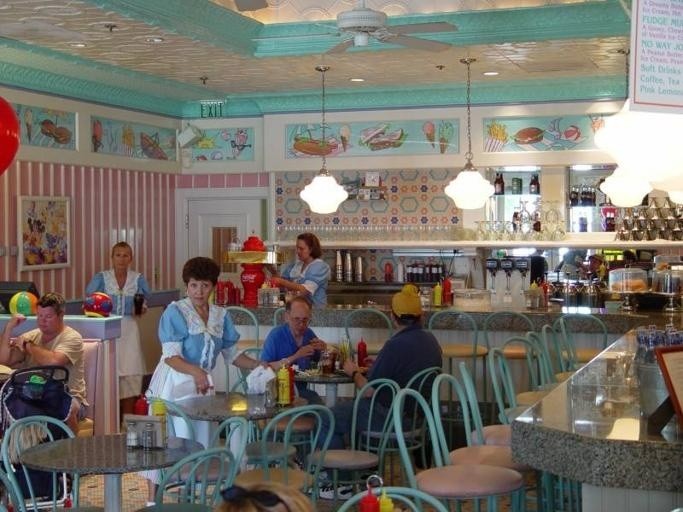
[289,317,312,324]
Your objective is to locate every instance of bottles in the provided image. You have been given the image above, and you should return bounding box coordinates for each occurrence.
[513,212,520,230]
[581,187,587,205]
[533,214,541,232]
[151,395,166,448]
[442,276,450,306]
[144,450,155,467]
[499,174,503,194]
[433,282,442,306]
[358,487,379,512]
[587,187,592,205]
[530,176,537,194]
[215,277,241,307]
[610,212,614,231]
[127,423,139,448]
[634,324,683,364]
[358,338,368,369]
[571,186,576,206]
[385,262,392,283]
[540,281,550,308]
[592,188,596,206]
[143,423,156,448]
[126,450,138,470]
[495,173,502,194]
[577,188,579,205]
[535,174,540,193]
[277,364,290,404]
[606,213,611,232]
[405,261,443,282]
[397,262,404,282]
[135,395,147,415]
[531,281,538,290]
[376,489,393,511]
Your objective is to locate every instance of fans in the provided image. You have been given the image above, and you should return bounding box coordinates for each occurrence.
[250,0,458,57]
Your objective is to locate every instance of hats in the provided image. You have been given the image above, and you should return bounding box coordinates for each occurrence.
[391,284,423,318]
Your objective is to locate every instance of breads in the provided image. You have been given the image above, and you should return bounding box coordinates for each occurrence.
[612,278,647,290]
[655,276,680,292]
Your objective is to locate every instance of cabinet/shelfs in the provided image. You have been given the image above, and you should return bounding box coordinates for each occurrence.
[565,165,649,233]
[486,165,544,240]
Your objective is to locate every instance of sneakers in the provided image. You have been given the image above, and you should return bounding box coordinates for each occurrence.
[309,484,352,499]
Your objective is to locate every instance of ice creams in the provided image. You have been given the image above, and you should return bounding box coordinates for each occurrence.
[423,122,436,150]
[340,124,351,152]
[25,109,33,142]
[440,122,454,152]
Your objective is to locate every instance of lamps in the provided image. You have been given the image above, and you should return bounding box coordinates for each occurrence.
[444,57,494,210]
[299,66,350,215]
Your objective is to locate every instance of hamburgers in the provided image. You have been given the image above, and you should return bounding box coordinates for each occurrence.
[510,128,548,145]
[52,126,73,144]
[39,120,55,137]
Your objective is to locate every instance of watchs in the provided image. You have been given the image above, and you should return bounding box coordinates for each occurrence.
[280,357,291,367]
[351,370,359,382]
[22,339,34,353]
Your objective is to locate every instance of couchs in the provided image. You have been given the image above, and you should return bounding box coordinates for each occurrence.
[0,337,103,434]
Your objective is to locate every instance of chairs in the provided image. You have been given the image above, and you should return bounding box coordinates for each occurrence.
[393,388,524,511]
[483,311,542,420]
[160,415,248,505]
[0,469,19,509]
[275,306,316,392]
[233,404,336,504]
[307,379,401,500]
[552,313,607,371]
[222,306,260,392]
[229,377,316,456]
[1,414,104,512]
[500,337,552,407]
[523,330,566,391]
[145,396,208,502]
[459,361,551,512]
[236,348,265,391]
[431,373,544,512]
[357,366,442,479]
[429,308,488,421]
[137,448,235,512]
[345,308,395,408]
[560,318,589,372]
[336,486,448,512]
[542,325,578,381]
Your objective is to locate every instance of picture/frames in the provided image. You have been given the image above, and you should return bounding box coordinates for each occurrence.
[646,346,683,437]
[16,196,72,273]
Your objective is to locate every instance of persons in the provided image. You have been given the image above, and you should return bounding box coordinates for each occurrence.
[317,285,442,499]
[268,232,331,307]
[577,254,604,280]
[262,296,328,412]
[1,292,89,437]
[84,242,151,422]
[213,482,313,512]
[142,257,272,507]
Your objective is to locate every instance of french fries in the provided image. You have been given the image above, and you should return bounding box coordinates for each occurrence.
[486,121,509,152]
